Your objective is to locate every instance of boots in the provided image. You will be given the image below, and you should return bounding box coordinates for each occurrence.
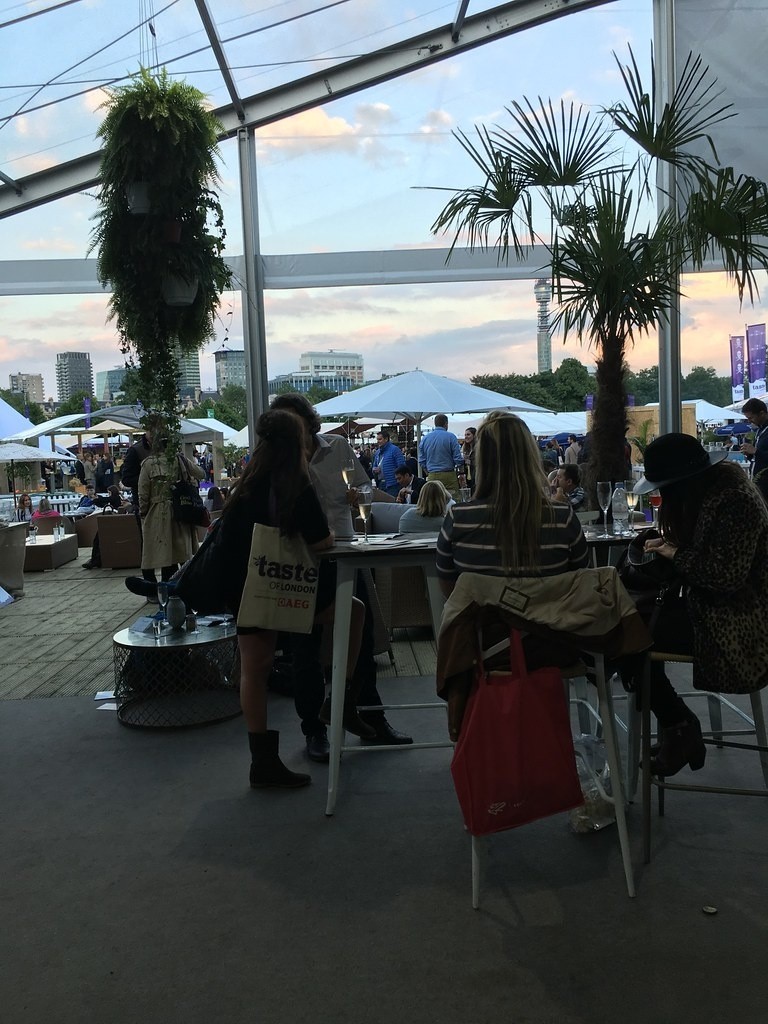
[246,729,312,789]
[320,666,372,737]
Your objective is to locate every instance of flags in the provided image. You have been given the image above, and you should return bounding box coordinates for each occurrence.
[747,324,766,397]
[731,336,744,401]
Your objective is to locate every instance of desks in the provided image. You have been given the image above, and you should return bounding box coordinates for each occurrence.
[314,511,656,817]
[0,521,30,593]
[23,533,78,571]
[112,613,243,731]
[59,512,88,523]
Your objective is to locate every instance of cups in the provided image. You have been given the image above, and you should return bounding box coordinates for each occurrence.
[53,528,59,541]
[401,493,408,504]
[716,442,722,451]
[459,488,472,502]
[30,531,36,544]
[710,442,714,451]
[60,524,65,538]
[153,621,161,639]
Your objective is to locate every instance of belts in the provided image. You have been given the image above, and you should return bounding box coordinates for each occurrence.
[443,468,454,472]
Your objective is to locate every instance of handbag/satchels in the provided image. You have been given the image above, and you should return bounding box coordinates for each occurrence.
[613,529,670,590]
[170,454,210,529]
[236,523,319,633]
[452,628,587,839]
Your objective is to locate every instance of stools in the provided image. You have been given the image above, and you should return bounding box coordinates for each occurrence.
[461,565,768,910]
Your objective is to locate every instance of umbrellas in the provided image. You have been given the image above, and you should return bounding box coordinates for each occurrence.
[313,370,558,478]
[683,399,748,437]
[0,443,78,508]
[712,422,757,436]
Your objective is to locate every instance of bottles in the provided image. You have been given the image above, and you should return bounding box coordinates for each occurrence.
[612,482,629,535]
[167,596,186,629]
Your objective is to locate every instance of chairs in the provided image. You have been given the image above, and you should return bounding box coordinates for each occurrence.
[32,510,141,569]
[196,509,223,543]
[354,489,454,667]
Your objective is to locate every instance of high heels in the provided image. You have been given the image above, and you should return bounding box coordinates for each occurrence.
[638,703,706,776]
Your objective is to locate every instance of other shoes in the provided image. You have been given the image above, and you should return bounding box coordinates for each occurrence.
[82,561,101,568]
[124,575,170,598]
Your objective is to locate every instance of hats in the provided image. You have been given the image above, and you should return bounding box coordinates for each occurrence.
[631,430,729,495]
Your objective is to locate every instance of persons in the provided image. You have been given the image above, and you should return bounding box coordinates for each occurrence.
[40,453,114,492]
[435,409,589,600]
[83,501,133,570]
[32,499,60,520]
[418,414,467,503]
[355,430,451,532]
[108,486,119,496]
[79,485,102,508]
[639,433,768,776]
[543,435,585,510]
[213,411,377,786]
[207,487,229,511]
[740,398,768,498]
[16,494,33,522]
[461,427,478,492]
[123,414,207,604]
[270,393,414,745]
[725,434,748,451]
[194,450,212,465]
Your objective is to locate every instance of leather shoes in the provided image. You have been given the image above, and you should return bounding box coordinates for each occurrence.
[360,717,412,747]
[307,735,331,760]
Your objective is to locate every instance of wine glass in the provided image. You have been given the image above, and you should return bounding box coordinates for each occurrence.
[648,490,661,533]
[190,609,202,635]
[220,613,231,626]
[597,482,615,539]
[157,585,170,626]
[358,492,372,546]
[626,480,641,537]
